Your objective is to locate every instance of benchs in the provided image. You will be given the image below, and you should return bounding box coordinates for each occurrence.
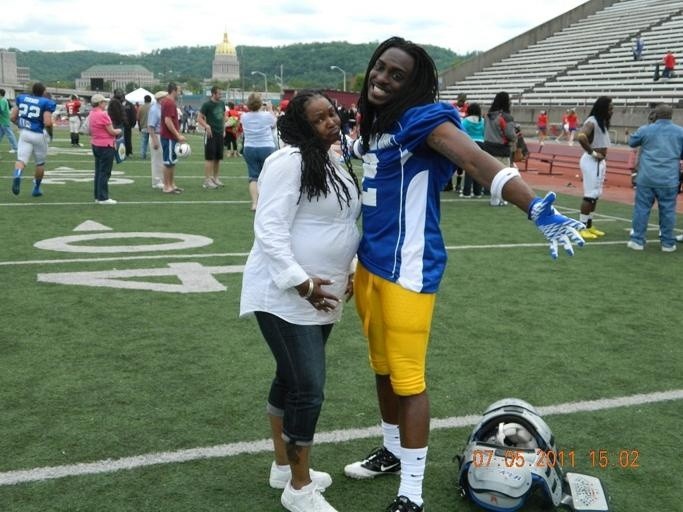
[434,0,683,109]
[523,142,637,176]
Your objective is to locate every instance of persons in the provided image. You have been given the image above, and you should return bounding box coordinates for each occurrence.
[67,83,196,203]
[0,82,56,195]
[446,92,528,197]
[635,35,644,61]
[339,35,586,512]
[198,85,295,211]
[627,105,683,251]
[663,50,676,77]
[537,108,577,146]
[339,103,360,139]
[577,96,614,239]
[239,89,361,512]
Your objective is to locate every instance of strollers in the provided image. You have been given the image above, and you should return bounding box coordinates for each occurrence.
[182,115,200,136]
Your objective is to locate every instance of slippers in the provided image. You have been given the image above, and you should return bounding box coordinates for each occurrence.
[163,188,184,194]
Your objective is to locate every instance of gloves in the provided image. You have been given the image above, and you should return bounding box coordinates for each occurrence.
[528,192,585,260]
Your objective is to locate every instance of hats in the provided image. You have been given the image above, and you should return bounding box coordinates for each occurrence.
[91,93,110,103]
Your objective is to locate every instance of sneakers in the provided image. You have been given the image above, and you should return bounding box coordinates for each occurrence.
[268,460,332,492]
[662,244,677,252]
[444,183,492,198]
[382,495,425,511]
[586,226,605,236]
[342,447,400,481]
[12,177,19,194]
[202,177,224,190]
[94,198,117,204]
[32,189,42,196]
[279,480,338,512]
[627,240,644,250]
[577,229,598,239]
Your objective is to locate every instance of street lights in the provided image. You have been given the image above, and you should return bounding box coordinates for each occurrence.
[157,69,176,95]
[330,65,346,93]
[250,70,268,94]
[55,79,61,106]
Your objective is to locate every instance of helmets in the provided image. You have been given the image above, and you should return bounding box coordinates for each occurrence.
[453,397,573,511]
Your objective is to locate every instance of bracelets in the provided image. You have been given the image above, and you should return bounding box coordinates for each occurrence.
[590,151,597,157]
[299,278,314,299]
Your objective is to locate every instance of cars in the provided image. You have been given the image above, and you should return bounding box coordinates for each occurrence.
[53,104,68,122]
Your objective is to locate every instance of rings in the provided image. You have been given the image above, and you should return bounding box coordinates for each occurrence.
[313,302,320,307]
[320,300,324,304]
